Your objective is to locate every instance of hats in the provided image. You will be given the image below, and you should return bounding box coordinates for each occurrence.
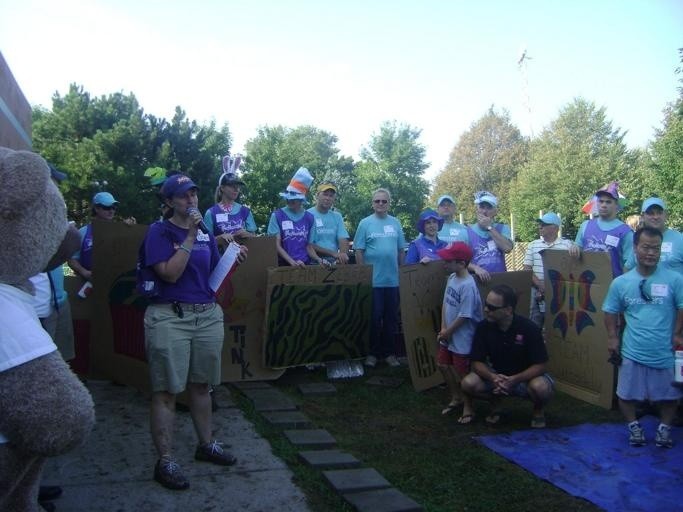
[417,207,444,233]
[162,174,200,195]
[642,197,665,212]
[438,195,455,205]
[596,182,618,200]
[50,165,66,180]
[222,173,245,186]
[317,180,338,193]
[279,167,314,205]
[94,192,120,207]
[535,212,560,225]
[474,192,496,207]
[437,241,472,260]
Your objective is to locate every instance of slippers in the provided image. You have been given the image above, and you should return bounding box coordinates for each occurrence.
[530,415,547,429]
[441,403,512,426]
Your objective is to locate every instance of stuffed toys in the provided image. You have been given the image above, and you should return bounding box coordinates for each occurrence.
[0,144,98,510]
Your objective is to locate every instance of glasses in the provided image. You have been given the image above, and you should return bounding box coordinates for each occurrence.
[322,181,338,188]
[485,301,504,311]
[373,200,387,203]
[639,279,652,301]
[285,190,301,196]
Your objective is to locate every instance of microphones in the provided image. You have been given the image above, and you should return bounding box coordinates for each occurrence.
[187,207,209,234]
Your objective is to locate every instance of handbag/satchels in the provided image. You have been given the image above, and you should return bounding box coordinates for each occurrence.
[136,243,161,297]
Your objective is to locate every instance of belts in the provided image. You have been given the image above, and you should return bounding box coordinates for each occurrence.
[167,300,216,313]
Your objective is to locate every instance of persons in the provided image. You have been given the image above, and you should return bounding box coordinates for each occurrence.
[29,161,78,376]
[69,191,136,283]
[136,156,407,489]
[404,184,682,448]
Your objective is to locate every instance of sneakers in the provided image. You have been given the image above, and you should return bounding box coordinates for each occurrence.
[195,442,236,465]
[655,426,673,449]
[385,355,400,368]
[628,424,647,445]
[366,356,377,368]
[154,458,189,490]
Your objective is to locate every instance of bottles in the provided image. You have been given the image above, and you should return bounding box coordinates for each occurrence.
[675,346,683,382]
[78,280,93,298]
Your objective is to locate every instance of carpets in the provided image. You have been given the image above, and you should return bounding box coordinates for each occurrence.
[471,413,680,512]
